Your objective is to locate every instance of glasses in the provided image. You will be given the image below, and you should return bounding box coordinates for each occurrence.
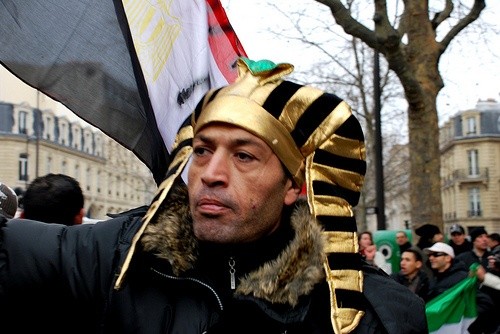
[427,252,448,257]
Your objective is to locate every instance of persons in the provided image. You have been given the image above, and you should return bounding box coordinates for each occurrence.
[470,234,500,291]
[1,57,428,334]
[456,226,493,270]
[396,231,412,270]
[432,224,472,257]
[358,231,386,273]
[20,173,85,226]
[424,242,495,334]
[415,224,440,250]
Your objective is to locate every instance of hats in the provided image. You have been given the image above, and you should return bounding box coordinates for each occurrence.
[450,224,465,235]
[114,56,366,334]
[470,228,488,241]
[487,234,500,243]
[423,242,455,259]
[0,183,18,219]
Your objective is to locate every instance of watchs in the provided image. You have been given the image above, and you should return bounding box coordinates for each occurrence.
[389,249,431,301]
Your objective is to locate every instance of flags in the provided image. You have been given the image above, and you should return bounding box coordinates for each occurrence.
[0,0,248,189]
